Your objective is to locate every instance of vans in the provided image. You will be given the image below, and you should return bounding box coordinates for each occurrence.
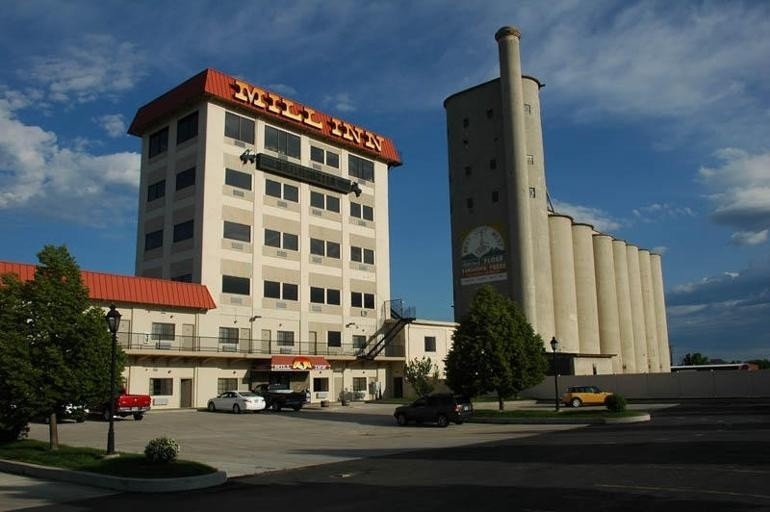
[393,392,474,428]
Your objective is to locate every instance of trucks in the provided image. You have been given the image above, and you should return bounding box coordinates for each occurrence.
[252,382,306,413]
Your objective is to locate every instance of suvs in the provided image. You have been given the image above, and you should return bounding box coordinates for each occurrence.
[560,385,614,408]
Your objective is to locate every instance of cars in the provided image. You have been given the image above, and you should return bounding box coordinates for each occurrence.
[207,389,267,414]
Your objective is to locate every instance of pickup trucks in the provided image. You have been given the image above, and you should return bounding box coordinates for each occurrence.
[67,375,152,423]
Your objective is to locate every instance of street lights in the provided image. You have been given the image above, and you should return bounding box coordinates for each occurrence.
[549,336,560,412]
[105,302,123,455]
